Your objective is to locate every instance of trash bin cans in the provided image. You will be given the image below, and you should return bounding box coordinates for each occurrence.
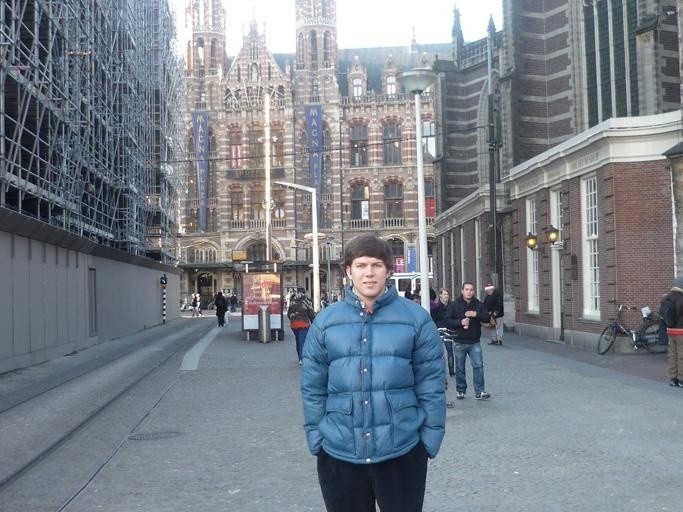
[257,310,271,343]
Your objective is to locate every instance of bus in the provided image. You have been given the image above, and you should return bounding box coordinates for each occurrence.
[385,270,434,301]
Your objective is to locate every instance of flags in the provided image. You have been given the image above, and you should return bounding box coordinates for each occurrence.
[393,10,415,45]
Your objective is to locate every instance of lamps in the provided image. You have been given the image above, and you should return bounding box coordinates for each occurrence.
[523,223,566,255]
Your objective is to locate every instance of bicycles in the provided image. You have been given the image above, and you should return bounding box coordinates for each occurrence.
[597,297,670,357]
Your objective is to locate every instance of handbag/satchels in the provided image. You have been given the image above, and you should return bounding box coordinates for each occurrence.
[659,301,676,327]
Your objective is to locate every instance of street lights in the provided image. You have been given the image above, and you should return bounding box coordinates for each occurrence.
[275,181,321,315]
[240,261,255,273]
[524,226,567,340]
[323,236,335,302]
[482,26,513,289]
[395,67,448,318]
[306,264,320,269]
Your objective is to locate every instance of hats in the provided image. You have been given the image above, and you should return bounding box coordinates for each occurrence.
[485,283,495,290]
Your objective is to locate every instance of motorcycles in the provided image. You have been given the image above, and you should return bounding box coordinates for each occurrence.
[180,298,194,313]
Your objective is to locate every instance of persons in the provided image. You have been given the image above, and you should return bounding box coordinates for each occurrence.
[658,277,683,388]
[301,233,446,511]
[189,288,237,327]
[406,280,504,401]
[286,286,338,365]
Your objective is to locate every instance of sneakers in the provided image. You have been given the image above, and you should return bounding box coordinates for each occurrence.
[487,339,502,345]
[475,392,490,400]
[457,391,464,399]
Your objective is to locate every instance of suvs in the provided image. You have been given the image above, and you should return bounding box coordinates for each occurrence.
[282,286,305,304]
